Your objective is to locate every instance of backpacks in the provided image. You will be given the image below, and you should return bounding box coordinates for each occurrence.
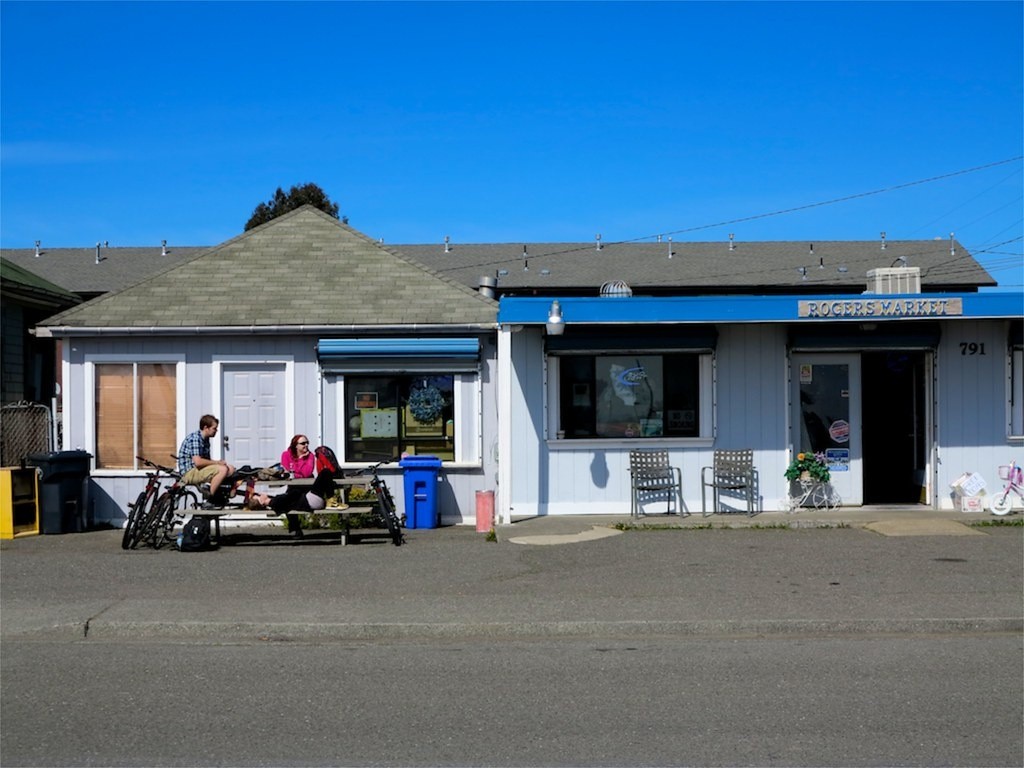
[315,446,344,479]
[180,518,211,553]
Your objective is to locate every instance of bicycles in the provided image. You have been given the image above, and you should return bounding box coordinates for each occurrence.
[121,456,173,550]
[345,460,407,547]
[129,474,198,550]
[989,459,1024,515]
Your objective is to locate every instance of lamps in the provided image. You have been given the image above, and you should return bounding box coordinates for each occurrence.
[545,300,566,336]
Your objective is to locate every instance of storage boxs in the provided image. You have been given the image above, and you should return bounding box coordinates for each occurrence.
[949,471,986,496]
[406,405,443,436]
[361,408,397,437]
[954,494,984,513]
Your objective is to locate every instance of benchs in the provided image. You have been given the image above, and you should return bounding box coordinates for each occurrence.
[174,506,373,546]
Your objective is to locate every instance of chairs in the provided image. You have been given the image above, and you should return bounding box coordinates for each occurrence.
[626,448,684,518]
[701,448,755,518]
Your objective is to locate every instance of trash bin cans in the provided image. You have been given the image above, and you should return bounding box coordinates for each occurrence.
[398,455,442,529]
[475,489,495,533]
[29,449,95,536]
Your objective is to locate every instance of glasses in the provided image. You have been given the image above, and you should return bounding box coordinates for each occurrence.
[297,441,310,446]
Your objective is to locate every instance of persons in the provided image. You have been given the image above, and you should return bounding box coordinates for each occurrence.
[179,414,235,508]
[247,468,349,515]
[281,434,315,539]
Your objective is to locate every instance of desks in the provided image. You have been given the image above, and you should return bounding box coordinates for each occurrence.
[174,477,374,545]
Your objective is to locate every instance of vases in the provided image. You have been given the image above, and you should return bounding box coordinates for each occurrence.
[800,470,812,479]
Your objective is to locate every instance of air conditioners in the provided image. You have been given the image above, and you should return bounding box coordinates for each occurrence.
[866,267,921,295]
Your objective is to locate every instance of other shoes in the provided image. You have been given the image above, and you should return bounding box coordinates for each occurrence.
[202,495,222,510]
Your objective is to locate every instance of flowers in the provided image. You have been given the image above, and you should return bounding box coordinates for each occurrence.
[785,452,830,483]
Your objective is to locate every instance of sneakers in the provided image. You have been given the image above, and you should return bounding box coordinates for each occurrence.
[325,496,349,510]
